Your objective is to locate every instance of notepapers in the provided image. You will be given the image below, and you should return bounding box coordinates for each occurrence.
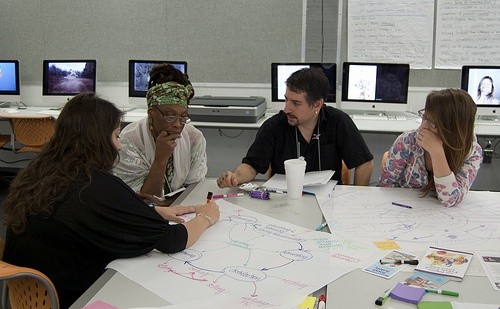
[390,282,424,305]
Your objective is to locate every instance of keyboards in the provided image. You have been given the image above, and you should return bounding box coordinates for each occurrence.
[0,107,19,114]
[352,113,406,120]
[474,120,500,126]
[38,109,61,116]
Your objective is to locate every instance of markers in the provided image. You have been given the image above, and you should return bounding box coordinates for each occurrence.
[380,260,419,265]
[392,202,413,209]
[213,193,243,198]
[317,294,326,309]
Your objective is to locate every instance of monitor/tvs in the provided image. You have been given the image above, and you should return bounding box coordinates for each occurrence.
[129,60,187,98]
[0,59,20,102]
[341,62,410,112]
[42,60,96,104]
[461,65,500,116]
[271,62,337,109]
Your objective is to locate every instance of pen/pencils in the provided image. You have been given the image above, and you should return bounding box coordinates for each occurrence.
[316,222,327,231]
[407,285,459,296]
[207,191,213,203]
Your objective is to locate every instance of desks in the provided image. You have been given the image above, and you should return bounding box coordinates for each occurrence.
[0,105,500,309]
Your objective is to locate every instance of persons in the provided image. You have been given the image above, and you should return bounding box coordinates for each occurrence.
[217,68,375,188]
[376,88,483,207]
[112,65,208,207]
[476,76,500,104]
[0,92,220,309]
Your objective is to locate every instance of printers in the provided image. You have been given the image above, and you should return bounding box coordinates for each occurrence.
[187,94,267,122]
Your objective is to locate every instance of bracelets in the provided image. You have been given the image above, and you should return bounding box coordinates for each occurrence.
[197,214,214,224]
[149,203,154,208]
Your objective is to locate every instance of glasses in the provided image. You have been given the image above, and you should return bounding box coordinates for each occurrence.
[417,108,438,128]
[155,105,191,124]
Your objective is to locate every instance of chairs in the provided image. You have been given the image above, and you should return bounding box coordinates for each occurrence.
[0,260,59,309]
[8,116,56,159]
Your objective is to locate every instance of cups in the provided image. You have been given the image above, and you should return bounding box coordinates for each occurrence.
[284,159,306,198]
